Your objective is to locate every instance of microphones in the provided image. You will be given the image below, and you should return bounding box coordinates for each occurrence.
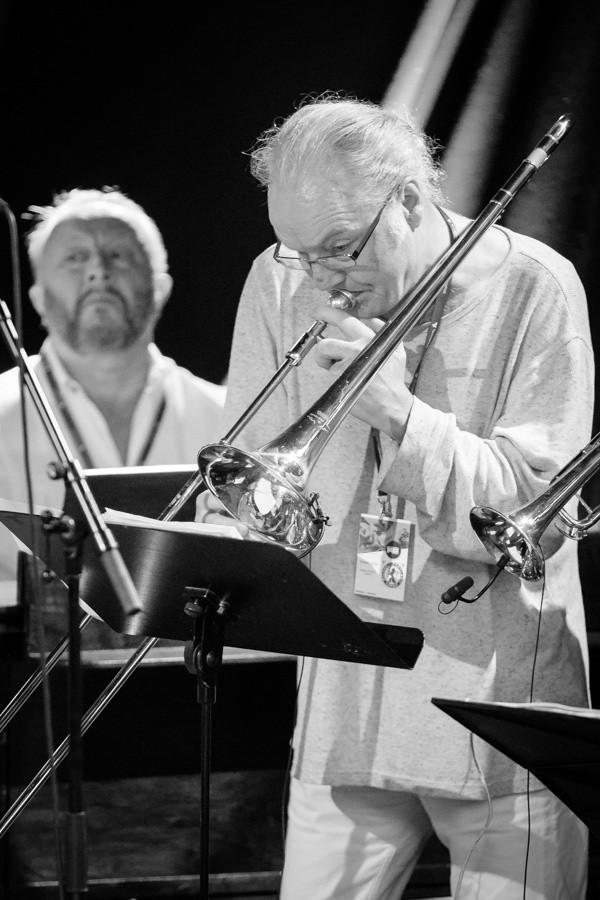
[441,565,502,604]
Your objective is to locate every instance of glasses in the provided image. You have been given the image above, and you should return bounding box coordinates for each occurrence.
[273,185,402,269]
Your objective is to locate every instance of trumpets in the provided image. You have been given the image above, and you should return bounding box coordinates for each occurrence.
[469,434,600,583]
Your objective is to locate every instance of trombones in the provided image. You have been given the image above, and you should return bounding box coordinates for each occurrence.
[0,106,575,836]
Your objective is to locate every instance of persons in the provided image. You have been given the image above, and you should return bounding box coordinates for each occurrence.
[194,99,597,900]
[1,187,229,603]
